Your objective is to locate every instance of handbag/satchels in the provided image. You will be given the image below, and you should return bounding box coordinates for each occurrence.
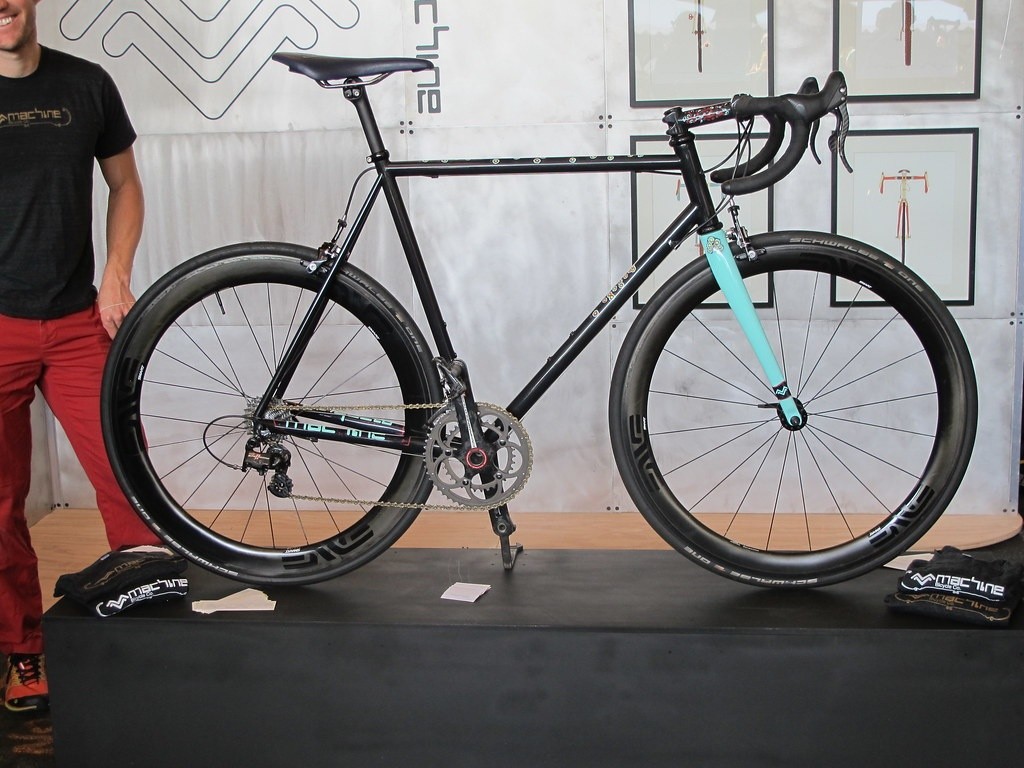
[884,545,1024,628]
[53,544,189,620]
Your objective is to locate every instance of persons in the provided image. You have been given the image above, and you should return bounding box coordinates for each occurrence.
[0,0,162,711]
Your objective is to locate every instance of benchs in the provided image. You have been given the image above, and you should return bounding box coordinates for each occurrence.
[39,545,1024,768]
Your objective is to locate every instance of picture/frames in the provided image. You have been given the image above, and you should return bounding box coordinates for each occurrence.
[631,0,983,311]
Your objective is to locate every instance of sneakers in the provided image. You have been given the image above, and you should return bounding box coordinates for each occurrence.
[4,652,50,712]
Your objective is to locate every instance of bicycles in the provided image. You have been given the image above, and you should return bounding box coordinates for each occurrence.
[97,51,980,588]
